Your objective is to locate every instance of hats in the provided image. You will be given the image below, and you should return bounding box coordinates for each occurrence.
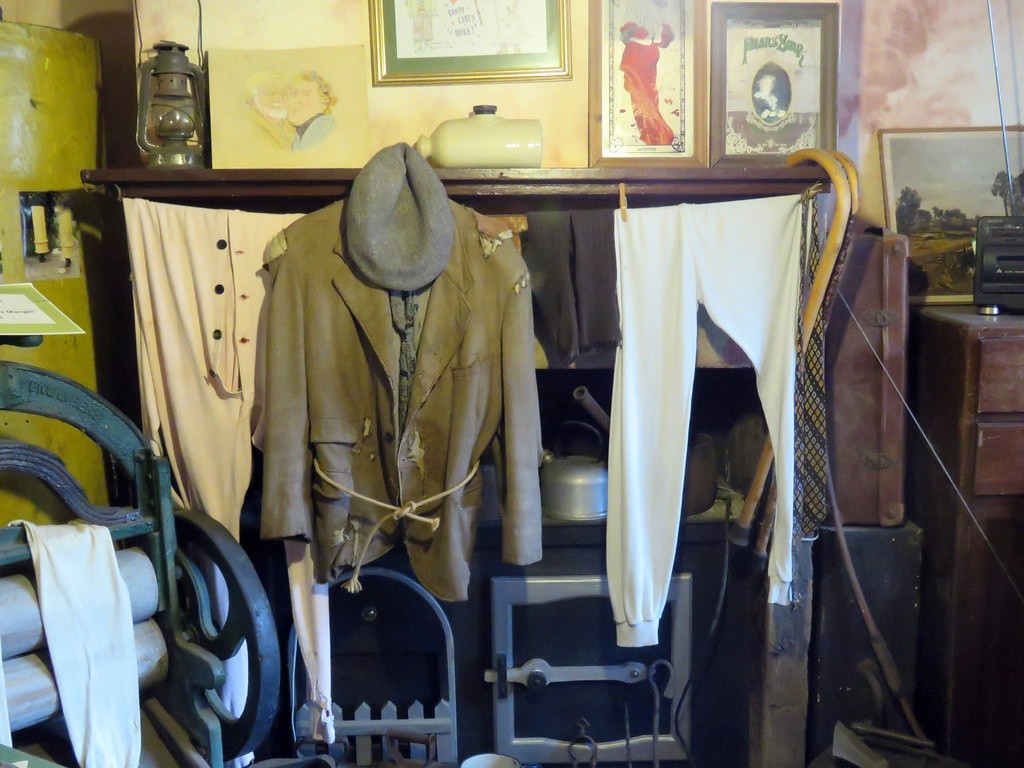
[341,140,454,293]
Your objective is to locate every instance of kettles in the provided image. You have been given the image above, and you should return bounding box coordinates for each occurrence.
[538,419,608,522]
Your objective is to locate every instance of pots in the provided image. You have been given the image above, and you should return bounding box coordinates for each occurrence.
[573,386,718,517]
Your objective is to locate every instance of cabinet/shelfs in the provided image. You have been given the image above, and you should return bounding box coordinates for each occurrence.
[908,303,1024,768]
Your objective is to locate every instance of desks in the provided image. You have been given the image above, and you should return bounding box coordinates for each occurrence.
[78,169,858,768]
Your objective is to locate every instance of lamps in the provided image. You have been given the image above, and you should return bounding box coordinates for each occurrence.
[135,0,212,169]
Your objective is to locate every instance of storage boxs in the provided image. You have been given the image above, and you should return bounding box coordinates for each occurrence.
[809,214,924,768]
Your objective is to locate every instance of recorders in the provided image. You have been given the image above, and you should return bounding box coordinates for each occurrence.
[969,214,1023,314]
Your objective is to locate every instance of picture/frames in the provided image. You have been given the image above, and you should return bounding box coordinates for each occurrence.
[589,0,710,168]
[709,2,839,167]
[368,0,573,88]
[877,126,1024,305]
